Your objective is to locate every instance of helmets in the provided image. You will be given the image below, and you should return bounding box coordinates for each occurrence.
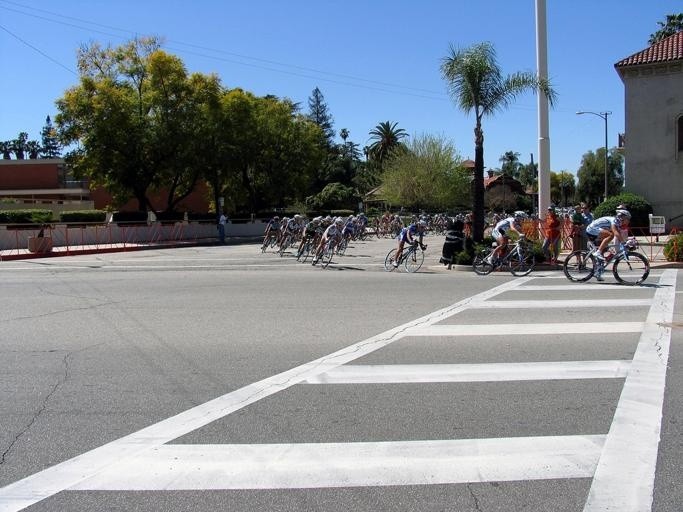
[418,220,427,226]
[273,214,304,222]
[311,213,366,226]
[514,211,526,218]
[616,209,632,219]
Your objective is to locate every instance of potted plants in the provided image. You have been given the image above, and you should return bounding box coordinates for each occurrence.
[28,213,64,252]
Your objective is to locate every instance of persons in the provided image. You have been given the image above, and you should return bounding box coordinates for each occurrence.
[263,200,629,283]
[219,212,228,242]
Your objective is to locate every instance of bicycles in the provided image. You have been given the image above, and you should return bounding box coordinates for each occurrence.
[382,240,426,273]
[410,221,447,237]
[259,220,405,269]
[472,235,536,279]
[563,237,650,286]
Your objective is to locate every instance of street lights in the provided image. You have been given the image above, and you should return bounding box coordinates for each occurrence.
[500,152,521,215]
[575,110,611,203]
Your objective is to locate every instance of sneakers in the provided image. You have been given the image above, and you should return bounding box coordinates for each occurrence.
[488,257,493,265]
[593,252,606,260]
[392,262,397,266]
